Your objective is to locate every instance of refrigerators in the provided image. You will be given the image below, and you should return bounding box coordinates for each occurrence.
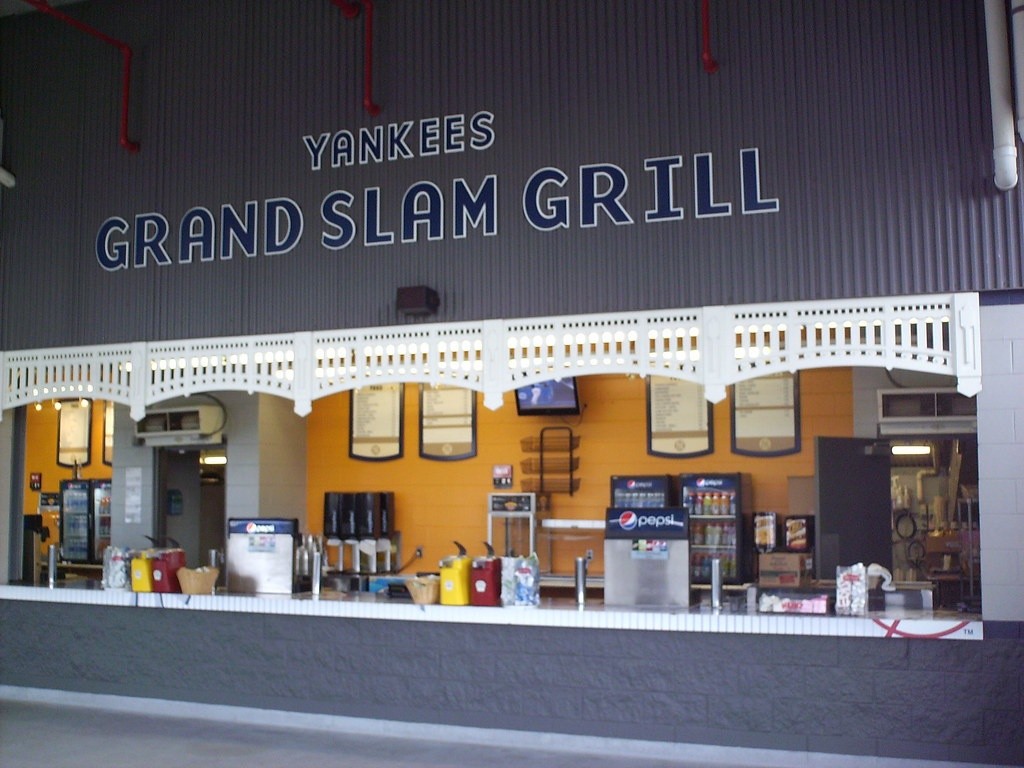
[609,474,675,510]
[680,472,753,587]
[59,476,113,563]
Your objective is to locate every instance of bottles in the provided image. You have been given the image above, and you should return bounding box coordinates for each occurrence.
[688,491,739,579]
[614,492,665,509]
[66,491,111,558]
[73,460,78,480]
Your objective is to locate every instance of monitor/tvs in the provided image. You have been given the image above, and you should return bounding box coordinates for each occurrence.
[514,375,580,415]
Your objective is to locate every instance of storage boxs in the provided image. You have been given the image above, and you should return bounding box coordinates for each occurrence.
[758,552,811,589]
[925,535,961,555]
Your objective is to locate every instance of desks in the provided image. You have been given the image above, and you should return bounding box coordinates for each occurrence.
[41,561,103,570]
[538,579,752,591]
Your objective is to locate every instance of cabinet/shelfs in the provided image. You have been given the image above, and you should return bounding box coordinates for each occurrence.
[519,425,581,497]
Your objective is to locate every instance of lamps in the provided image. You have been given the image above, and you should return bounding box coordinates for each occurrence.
[396,285,440,315]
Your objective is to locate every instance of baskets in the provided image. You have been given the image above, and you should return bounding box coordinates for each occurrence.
[175,565,220,594]
[404,574,440,605]
